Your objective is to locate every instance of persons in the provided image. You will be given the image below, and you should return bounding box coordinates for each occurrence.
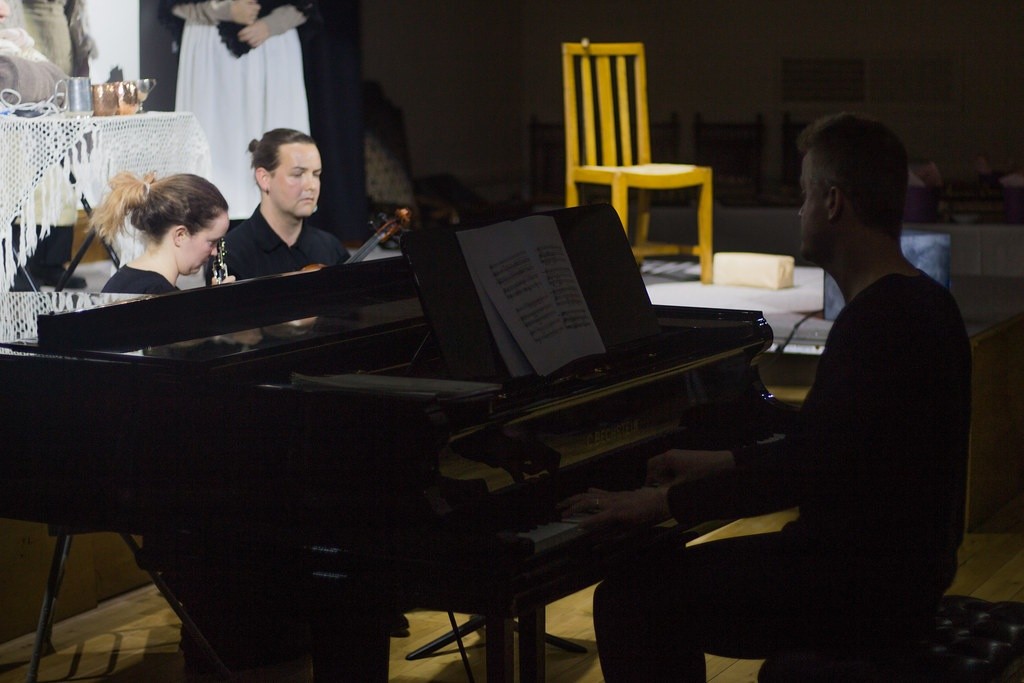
[169,0,325,239]
[82,170,263,361]
[218,128,353,281]
[544,111,974,683]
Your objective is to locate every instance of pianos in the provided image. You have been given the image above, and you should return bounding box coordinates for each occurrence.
[0,196,811,683]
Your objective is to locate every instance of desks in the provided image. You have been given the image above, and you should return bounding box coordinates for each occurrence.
[0,110,222,334]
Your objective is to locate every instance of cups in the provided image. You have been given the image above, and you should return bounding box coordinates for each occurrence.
[91,81,139,117]
[54,76,95,120]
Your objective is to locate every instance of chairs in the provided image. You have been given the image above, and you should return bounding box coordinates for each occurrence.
[614,112,690,206]
[692,111,778,207]
[782,114,812,206]
[526,112,610,203]
[561,38,713,285]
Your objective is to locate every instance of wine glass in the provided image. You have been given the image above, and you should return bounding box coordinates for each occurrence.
[137,78,150,114]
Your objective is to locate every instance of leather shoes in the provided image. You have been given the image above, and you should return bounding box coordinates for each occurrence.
[26,265,87,289]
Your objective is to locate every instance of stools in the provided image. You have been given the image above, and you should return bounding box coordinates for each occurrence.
[143,536,311,672]
[757,594,1024,683]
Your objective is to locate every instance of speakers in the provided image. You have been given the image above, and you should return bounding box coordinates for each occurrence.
[823,227,951,320]
